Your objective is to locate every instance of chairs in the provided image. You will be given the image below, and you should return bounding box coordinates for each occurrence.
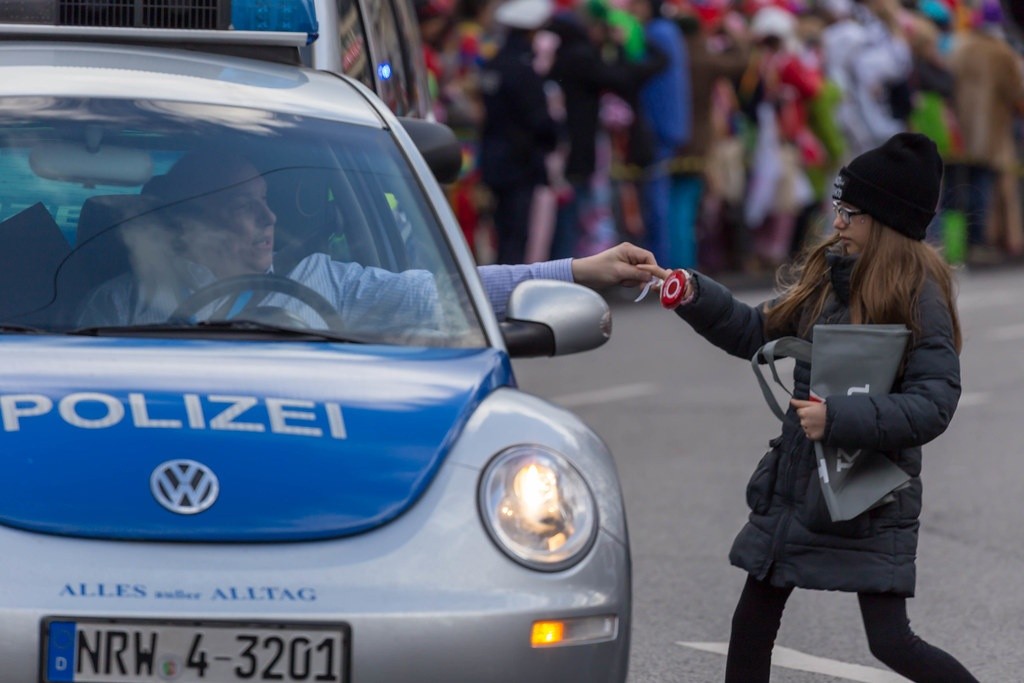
[102,166,329,280]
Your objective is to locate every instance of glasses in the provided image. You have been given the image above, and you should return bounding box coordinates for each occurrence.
[832,200,865,224]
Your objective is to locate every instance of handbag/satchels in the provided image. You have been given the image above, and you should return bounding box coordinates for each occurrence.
[751,323,914,523]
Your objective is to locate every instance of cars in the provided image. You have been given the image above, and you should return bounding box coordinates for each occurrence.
[0,0,631,682]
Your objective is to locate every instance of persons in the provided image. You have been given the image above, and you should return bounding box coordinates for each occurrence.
[412,0,1024,301]
[74,145,658,332]
[636,132,980,683]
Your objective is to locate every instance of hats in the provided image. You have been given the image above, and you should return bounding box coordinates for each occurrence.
[831,133,943,239]
